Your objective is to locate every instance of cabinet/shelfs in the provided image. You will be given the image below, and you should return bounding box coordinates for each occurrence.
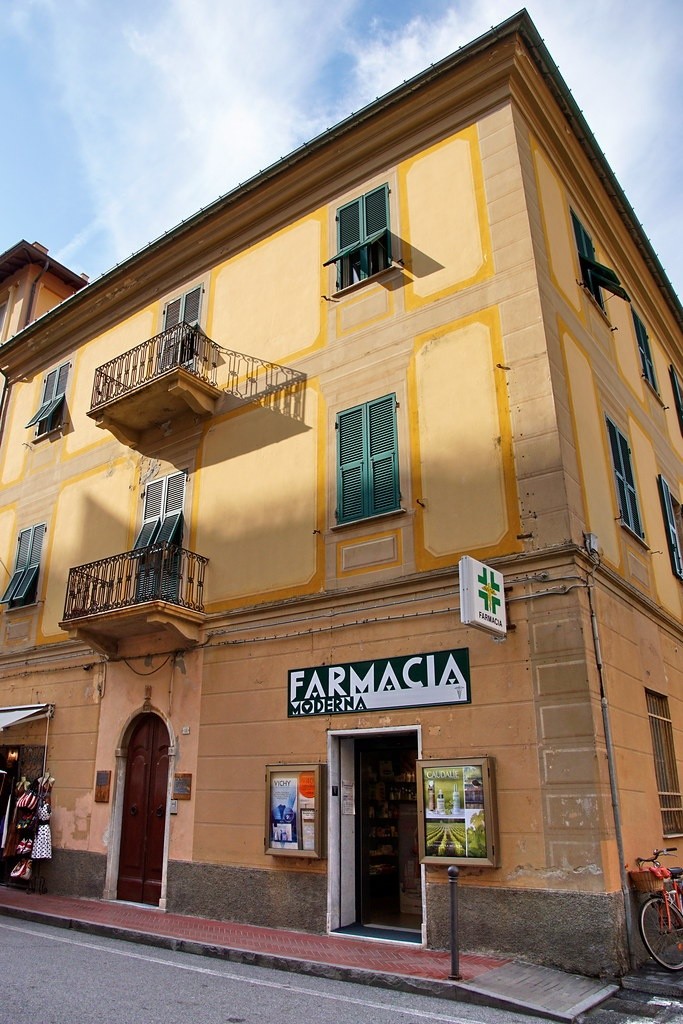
[365,774,417,877]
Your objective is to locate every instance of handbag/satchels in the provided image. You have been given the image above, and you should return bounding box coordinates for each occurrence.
[16,838,33,855]
[16,812,38,832]
[10,859,32,881]
[16,790,40,810]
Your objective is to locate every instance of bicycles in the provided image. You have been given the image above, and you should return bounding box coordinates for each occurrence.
[629,847,683,972]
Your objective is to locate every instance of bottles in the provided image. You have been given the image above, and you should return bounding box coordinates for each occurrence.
[401,771,415,782]
[388,786,411,801]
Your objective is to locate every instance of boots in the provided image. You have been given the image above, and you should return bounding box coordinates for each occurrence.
[25,876,49,895]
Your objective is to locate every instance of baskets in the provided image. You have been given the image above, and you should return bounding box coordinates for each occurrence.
[628,870,664,894]
